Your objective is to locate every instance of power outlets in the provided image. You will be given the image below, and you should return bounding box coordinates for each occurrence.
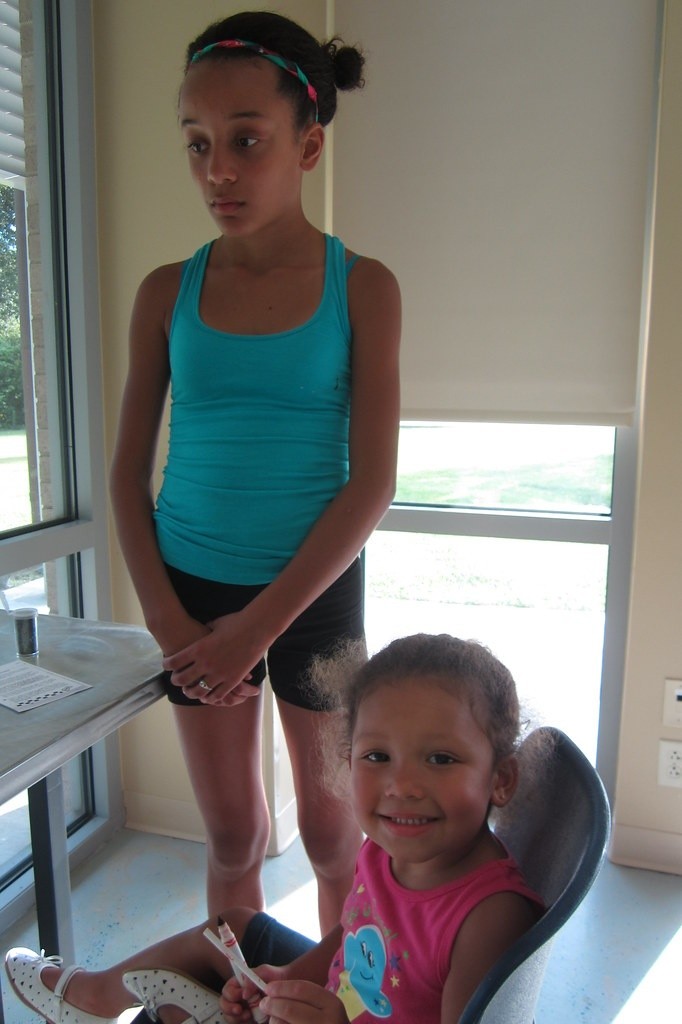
[657,738,681,788]
[660,678,682,729]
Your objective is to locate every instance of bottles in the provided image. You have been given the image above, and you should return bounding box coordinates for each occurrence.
[12,605,38,657]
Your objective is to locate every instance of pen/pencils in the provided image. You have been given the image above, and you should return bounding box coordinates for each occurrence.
[203,927,268,996]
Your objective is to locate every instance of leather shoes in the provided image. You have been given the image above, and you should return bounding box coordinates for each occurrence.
[121,963,229,1024]
[4,946,119,1024]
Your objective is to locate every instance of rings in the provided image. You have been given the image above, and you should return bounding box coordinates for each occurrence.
[196,682,216,695]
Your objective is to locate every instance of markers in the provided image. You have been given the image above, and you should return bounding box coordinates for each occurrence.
[217,916,270,1024]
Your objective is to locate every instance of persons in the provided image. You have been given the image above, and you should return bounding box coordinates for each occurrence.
[113,2,411,940]
[11,627,563,1024]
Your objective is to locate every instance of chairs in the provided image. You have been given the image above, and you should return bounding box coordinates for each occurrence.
[128,723,612,1024]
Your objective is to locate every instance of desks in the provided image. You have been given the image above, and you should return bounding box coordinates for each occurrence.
[0,607,169,1022]
